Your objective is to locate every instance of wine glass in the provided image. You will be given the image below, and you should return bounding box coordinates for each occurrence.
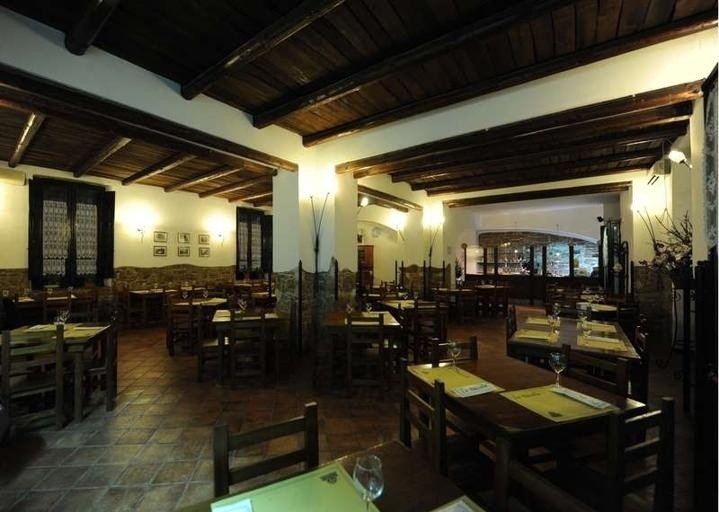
[547,301,593,346]
[549,352,568,390]
[238,299,247,312]
[366,303,372,316]
[403,294,408,304]
[353,454,385,511]
[585,296,600,307]
[448,341,461,368]
[182,291,189,300]
[203,291,208,303]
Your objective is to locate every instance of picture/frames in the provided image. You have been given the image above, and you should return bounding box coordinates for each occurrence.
[150,230,212,261]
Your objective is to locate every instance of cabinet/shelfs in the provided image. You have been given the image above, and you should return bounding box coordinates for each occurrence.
[357,242,377,274]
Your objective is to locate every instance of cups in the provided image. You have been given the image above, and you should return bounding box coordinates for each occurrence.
[414,292,418,300]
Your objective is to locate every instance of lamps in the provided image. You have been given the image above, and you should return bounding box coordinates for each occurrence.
[667,148,692,172]
[427,217,443,268]
[305,186,337,272]
[356,196,371,216]
